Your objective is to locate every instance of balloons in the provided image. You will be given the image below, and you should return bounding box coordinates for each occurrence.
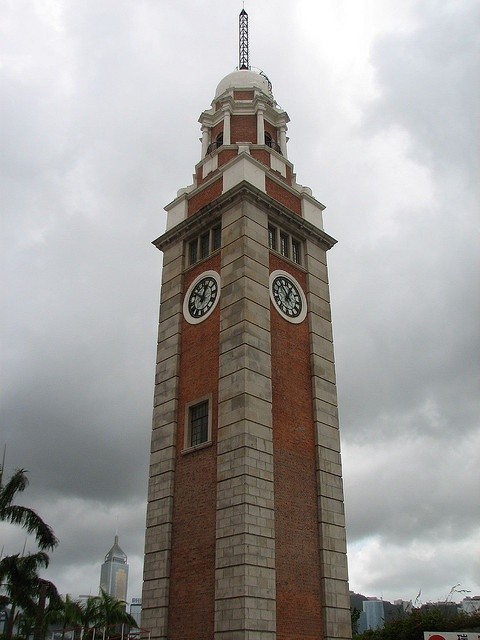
[182,269,222,326]
[268,267,309,326]
